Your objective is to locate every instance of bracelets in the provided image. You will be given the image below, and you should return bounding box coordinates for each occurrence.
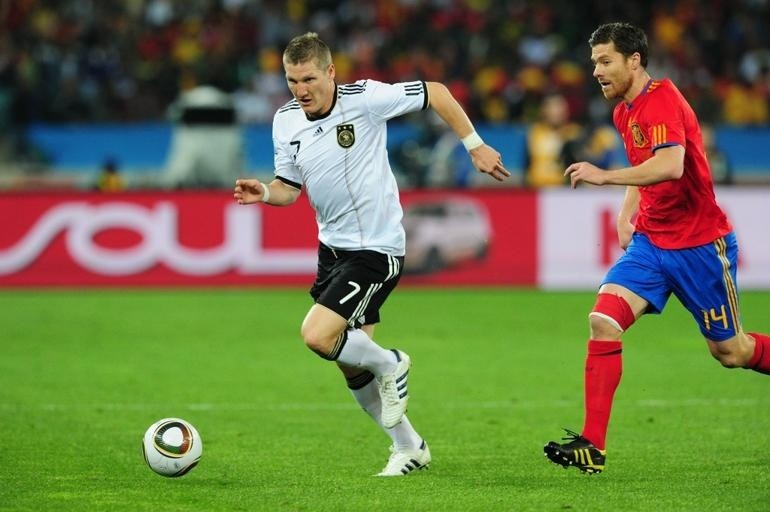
[230,30,513,482]
[259,183,270,203]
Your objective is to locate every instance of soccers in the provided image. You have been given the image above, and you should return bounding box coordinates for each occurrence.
[142,417,203,478]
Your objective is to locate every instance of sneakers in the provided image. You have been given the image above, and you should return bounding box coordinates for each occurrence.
[376,347,411,430]
[542,431,608,474]
[373,435,432,477]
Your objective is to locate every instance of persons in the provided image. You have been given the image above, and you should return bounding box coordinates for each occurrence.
[0,1,769,184]
[538,19,769,476]
